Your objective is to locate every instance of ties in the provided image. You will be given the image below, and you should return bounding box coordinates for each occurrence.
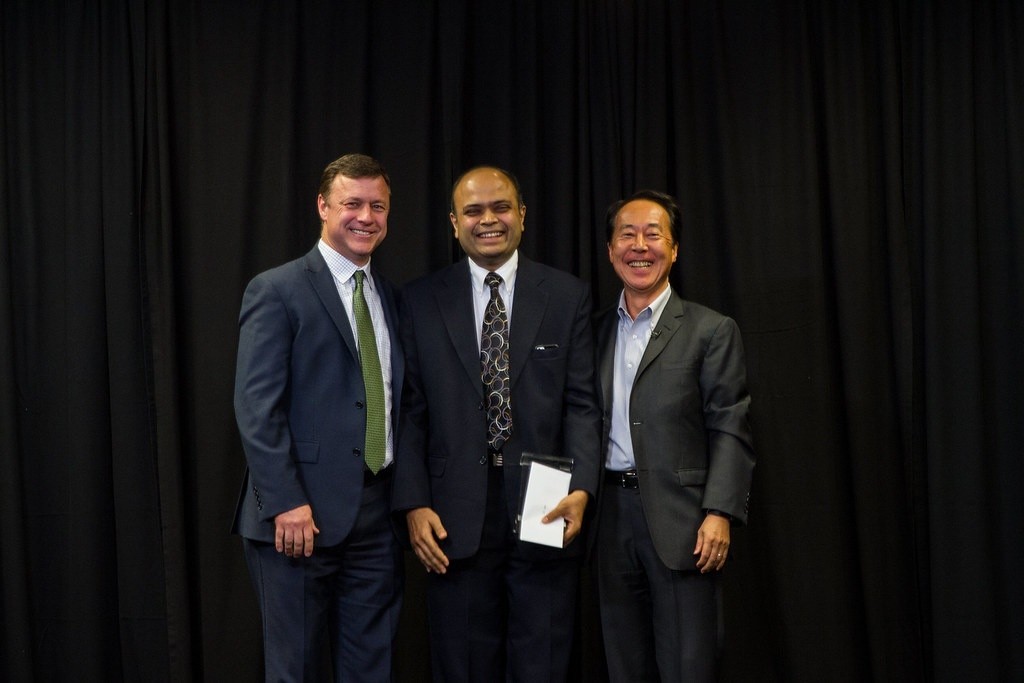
[479,272,515,455]
[353,270,386,475]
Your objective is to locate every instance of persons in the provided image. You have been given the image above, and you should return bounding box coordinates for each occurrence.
[230,154,406,683]
[399,165,602,683]
[591,190,755,683]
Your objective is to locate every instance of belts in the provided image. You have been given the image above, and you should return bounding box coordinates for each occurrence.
[489,453,504,467]
[605,471,639,490]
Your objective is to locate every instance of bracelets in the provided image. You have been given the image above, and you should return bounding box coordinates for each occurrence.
[708,509,735,524]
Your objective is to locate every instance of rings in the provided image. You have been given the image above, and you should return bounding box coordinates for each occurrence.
[718,554,723,557]
